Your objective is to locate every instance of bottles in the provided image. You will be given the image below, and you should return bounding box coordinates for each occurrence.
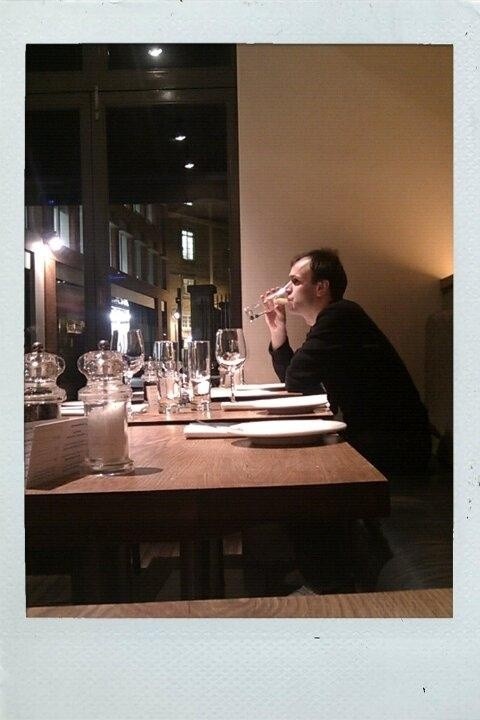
[76,339,135,477]
[25,341,67,420]
[143,354,158,382]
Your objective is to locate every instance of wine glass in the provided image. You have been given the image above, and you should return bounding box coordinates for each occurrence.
[214,328,246,403]
[112,328,145,416]
[154,341,185,414]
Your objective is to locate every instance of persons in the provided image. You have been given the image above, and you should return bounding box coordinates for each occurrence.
[260,243,434,596]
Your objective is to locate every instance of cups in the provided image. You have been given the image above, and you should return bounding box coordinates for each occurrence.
[244,290,289,324]
[187,340,211,412]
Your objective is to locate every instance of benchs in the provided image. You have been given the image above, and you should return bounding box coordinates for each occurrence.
[374,309,452,600]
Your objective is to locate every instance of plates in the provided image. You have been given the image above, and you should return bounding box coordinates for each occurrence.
[252,392,328,413]
[258,382,285,389]
[227,420,347,443]
